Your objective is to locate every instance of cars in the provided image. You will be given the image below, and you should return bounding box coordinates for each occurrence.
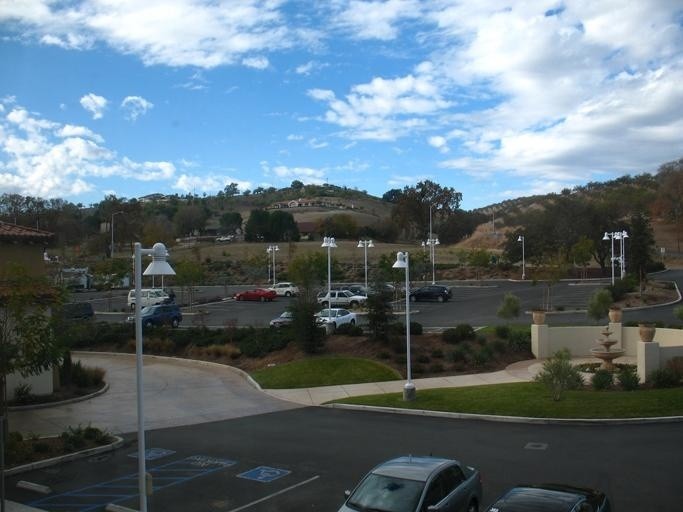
[236,288,275,303]
[270,308,356,329]
[337,453,482,512]
[318,284,394,309]
[484,482,610,512]
[271,282,299,297]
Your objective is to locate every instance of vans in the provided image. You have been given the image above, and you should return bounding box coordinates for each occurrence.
[127,288,169,310]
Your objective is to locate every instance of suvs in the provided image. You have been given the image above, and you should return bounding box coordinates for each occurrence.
[410,286,452,303]
[128,305,182,329]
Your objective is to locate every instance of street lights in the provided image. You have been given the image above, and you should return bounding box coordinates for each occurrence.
[393,251,414,388]
[110,211,125,259]
[131,240,176,512]
[518,236,526,279]
[358,239,375,295]
[602,230,629,285]
[426,238,441,286]
[322,236,338,322]
[268,246,280,284]
